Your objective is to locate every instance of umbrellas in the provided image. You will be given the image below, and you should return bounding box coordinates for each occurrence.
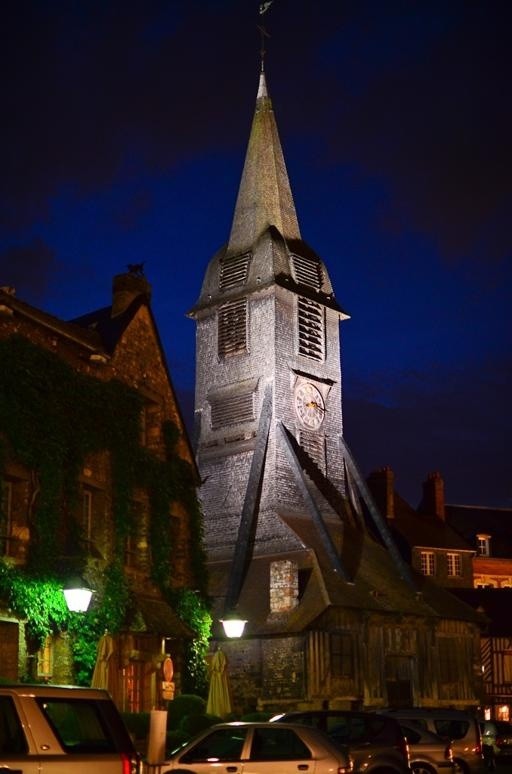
[89,626,127,716]
[204,645,239,722]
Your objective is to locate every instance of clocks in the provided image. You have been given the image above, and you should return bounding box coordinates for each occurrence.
[294,383,325,431]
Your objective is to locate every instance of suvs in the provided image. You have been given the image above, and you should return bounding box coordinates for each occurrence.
[0,684,145,774]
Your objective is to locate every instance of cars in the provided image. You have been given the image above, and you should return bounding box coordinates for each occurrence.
[157,719,352,774]
[252,707,512,774]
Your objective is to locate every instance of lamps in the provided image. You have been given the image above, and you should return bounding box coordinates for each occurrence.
[61,575,96,612]
[218,607,249,638]
